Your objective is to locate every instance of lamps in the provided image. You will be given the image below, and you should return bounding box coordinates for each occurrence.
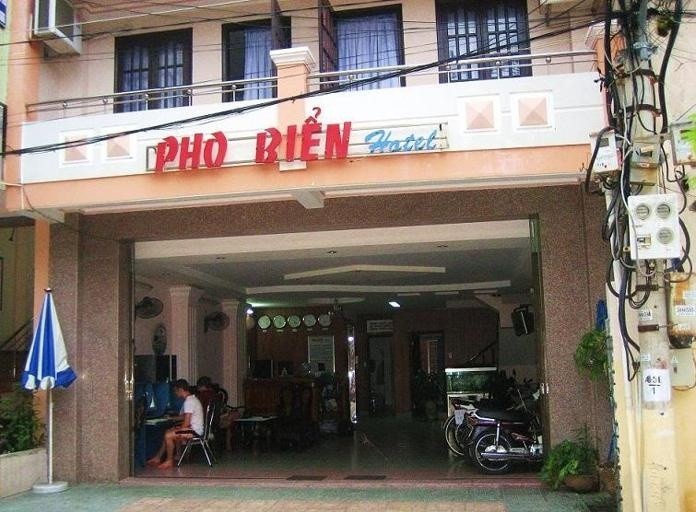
[241,302,253,315]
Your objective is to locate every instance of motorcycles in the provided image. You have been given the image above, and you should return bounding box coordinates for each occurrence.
[442,369,544,475]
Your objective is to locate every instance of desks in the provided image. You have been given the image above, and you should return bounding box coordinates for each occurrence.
[233,415,277,460]
[134,417,185,468]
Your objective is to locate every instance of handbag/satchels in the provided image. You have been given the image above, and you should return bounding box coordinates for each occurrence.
[219,409,240,428]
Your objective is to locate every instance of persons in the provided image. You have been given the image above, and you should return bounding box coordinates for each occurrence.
[172,375,219,461]
[146,378,203,470]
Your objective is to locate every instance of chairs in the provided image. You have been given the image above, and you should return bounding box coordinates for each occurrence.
[175,392,223,469]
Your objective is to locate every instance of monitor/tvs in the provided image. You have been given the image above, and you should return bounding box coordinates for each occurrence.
[254,360,274,379]
[511,309,534,336]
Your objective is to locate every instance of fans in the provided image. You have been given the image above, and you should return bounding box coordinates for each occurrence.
[136,296,163,319]
[204,311,229,333]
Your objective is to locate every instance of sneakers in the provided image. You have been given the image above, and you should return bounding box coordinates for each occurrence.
[158,462,173,470]
[147,458,160,464]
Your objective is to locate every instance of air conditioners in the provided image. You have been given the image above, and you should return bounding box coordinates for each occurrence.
[34,0,82,55]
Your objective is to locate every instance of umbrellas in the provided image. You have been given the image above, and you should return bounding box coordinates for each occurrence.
[19,288,76,484]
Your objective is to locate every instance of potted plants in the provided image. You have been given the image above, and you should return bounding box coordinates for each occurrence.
[538,422,600,495]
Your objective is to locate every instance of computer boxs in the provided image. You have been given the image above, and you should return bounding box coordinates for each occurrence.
[157,354,176,382]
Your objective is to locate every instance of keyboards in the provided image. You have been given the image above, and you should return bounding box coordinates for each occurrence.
[147,419,168,423]
